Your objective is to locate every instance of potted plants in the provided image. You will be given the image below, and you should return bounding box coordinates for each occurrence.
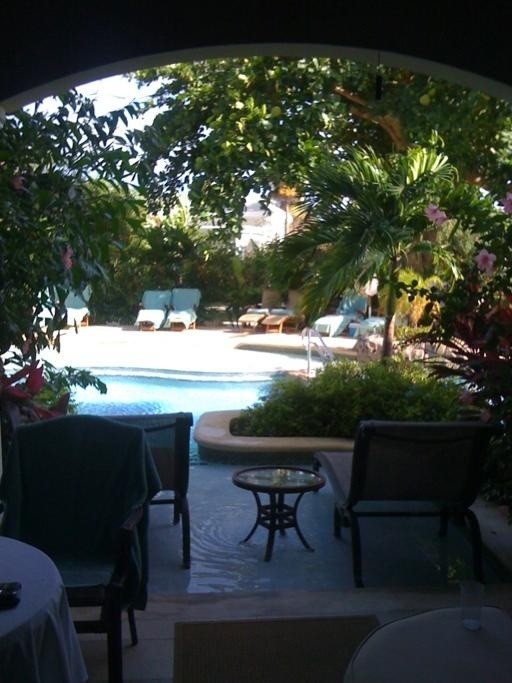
[446,554,484,631]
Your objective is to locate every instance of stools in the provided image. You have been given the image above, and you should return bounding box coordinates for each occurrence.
[343,603,510,682]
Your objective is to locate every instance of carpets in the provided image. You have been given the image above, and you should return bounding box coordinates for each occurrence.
[170,613,380,683]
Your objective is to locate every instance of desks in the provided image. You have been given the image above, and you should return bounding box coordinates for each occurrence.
[0,535,65,682]
[231,464,325,561]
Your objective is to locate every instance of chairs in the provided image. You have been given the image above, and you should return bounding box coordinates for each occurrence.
[312,293,368,335]
[311,419,497,588]
[237,289,306,334]
[134,287,200,331]
[31,282,92,327]
[7,410,193,683]
